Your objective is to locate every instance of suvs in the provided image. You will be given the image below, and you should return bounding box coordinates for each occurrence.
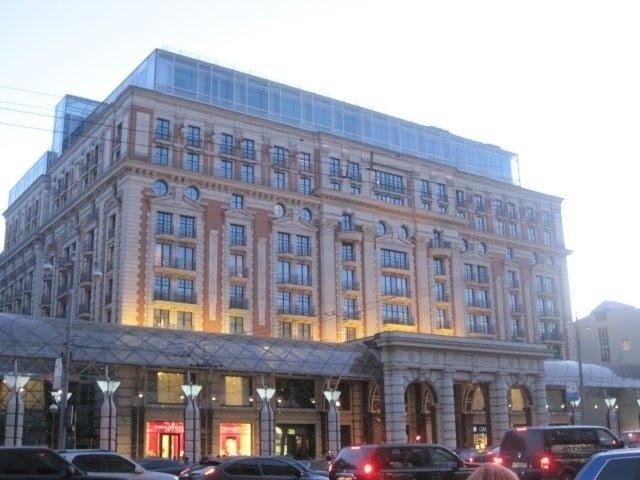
[500,426,627,479]
[328,444,478,479]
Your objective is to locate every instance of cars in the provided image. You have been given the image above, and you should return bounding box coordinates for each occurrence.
[575,448,639,479]
[455,443,501,468]
[621,431,640,448]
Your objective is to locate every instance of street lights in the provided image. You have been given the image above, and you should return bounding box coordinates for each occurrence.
[570,397,580,426]
[605,397,621,438]
[181,384,203,465]
[97,379,120,451]
[3,360,29,446]
[323,389,341,462]
[256,387,277,458]
[50,389,72,450]
